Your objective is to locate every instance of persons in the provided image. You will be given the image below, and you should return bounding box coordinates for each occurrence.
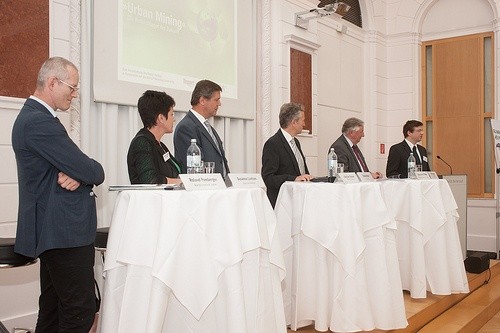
[261,102,314,211]
[329,118,383,180]
[12,56,105,333]
[127,90,187,185]
[174,80,232,187]
[386,120,430,178]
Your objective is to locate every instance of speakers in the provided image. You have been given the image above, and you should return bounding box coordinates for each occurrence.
[463,253,490,274]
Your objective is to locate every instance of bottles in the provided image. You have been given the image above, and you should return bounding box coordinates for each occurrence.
[186,139,201,174]
[408,153,416,179]
[328,148,337,183]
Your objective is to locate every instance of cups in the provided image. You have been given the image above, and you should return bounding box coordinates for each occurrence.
[337,163,344,173]
[416,165,422,172]
[200,161,205,173]
[205,162,215,174]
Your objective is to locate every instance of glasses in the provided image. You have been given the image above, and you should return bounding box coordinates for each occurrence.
[58,80,79,91]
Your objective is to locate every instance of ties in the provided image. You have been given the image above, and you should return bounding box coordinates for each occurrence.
[204,121,226,180]
[352,145,368,172]
[413,146,421,171]
[290,140,305,175]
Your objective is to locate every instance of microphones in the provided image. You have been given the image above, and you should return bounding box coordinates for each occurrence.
[436,155,452,175]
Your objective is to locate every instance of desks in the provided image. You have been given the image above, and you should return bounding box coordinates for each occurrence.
[279,181,388,331]
[113,185,269,332]
[388,178,446,293]
